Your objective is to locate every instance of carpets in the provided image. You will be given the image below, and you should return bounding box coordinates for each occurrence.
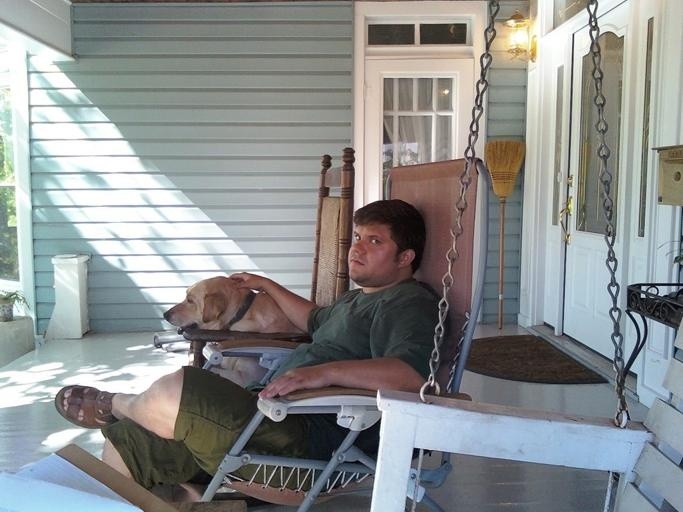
[464,333,611,384]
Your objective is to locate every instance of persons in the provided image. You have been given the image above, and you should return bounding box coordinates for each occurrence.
[53,200,451,511]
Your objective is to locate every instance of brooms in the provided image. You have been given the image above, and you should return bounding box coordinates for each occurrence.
[483,139,526,330]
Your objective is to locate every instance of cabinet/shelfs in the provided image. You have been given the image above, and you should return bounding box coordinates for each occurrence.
[622,282,683,420]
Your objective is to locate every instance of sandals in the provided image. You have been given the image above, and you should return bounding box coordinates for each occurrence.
[53,384,119,431]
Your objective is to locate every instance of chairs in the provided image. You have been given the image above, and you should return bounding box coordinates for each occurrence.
[368,314,683,512]
[53,157,489,512]
[178,147,355,366]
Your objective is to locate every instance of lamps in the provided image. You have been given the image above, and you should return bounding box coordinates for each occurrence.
[503,9,538,63]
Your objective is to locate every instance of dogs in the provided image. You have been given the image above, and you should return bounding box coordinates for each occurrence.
[163,276,309,390]
